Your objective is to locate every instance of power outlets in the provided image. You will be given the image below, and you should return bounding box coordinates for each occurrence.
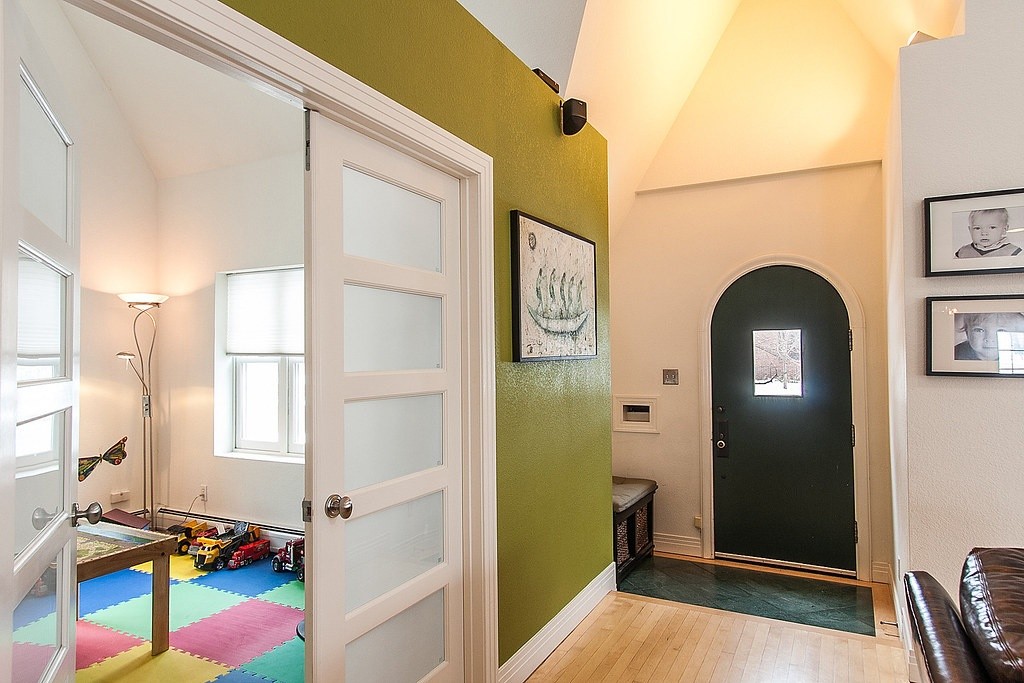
[199,485,207,502]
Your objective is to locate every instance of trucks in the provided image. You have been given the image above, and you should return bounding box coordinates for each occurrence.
[166,520,207,556]
[273,536,305,582]
[188,523,270,572]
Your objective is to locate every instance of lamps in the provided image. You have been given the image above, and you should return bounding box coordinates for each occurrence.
[115,294,170,536]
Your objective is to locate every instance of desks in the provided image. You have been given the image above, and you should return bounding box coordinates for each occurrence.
[77,518,179,656]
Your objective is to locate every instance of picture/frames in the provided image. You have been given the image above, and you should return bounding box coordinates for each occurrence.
[923,187,1024,277]
[926,294,1024,378]
[509,209,598,362]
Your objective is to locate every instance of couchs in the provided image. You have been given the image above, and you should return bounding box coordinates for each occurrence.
[904,547,1024,683]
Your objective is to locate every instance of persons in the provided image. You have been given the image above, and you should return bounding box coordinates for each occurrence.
[950,209,1022,258]
[952,313,1024,361]
